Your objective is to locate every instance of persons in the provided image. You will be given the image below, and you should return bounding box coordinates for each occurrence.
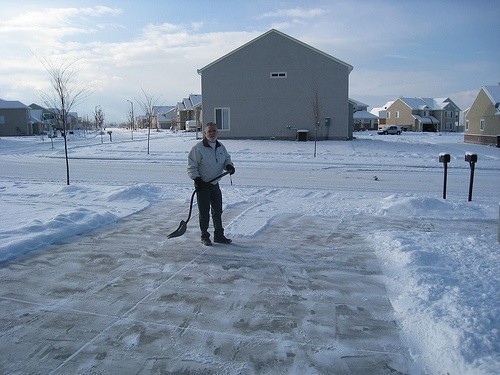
[187,122,235,246]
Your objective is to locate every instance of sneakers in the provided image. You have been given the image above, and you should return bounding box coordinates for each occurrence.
[201,236,212,245]
[214,235,232,243]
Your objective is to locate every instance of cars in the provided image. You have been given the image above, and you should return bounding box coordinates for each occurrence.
[377,125,402,135]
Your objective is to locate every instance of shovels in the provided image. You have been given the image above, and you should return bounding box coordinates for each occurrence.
[166,171,230,238]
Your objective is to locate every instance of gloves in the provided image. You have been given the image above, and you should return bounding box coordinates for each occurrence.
[227,165,235,175]
[195,177,207,188]
